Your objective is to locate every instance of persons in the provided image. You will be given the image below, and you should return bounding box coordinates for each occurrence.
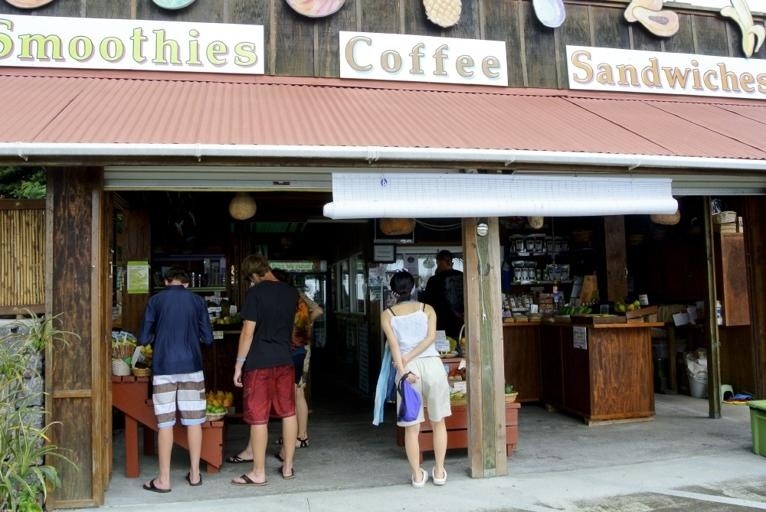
[379,270,448,487]
[423,248,467,343]
[224,267,324,464]
[231,254,300,486]
[135,264,216,493]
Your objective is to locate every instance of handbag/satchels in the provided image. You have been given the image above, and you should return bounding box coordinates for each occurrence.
[397,372,421,422]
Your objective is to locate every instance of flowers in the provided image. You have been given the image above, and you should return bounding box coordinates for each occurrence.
[133,343,153,368]
[205,389,232,413]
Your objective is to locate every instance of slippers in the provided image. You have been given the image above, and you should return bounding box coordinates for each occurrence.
[412,468,429,488]
[143,479,171,493]
[432,465,447,485]
[186,472,202,487]
[228,454,255,463]
[231,474,269,486]
[279,465,296,480]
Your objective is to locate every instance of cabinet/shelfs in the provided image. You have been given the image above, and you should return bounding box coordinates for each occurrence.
[499,217,665,421]
[392,357,522,465]
[150,253,236,332]
[112,374,225,477]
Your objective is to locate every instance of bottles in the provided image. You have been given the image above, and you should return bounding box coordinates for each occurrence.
[187,258,229,286]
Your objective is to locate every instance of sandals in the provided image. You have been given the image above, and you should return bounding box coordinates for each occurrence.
[273,436,310,461]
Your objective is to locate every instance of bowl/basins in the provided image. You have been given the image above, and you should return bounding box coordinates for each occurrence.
[202,407,228,421]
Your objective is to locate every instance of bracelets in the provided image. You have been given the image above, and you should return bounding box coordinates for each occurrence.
[235,357,246,363]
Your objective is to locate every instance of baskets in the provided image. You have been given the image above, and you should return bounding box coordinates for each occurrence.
[113,359,131,377]
[131,346,152,377]
[206,407,228,422]
[459,324,466,357]
[505,391,518,403]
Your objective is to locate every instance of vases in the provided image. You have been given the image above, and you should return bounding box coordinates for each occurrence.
[205,411,229,422]
[132,368,150,377]
[111,357,130,376]
[112,331,138,356]
